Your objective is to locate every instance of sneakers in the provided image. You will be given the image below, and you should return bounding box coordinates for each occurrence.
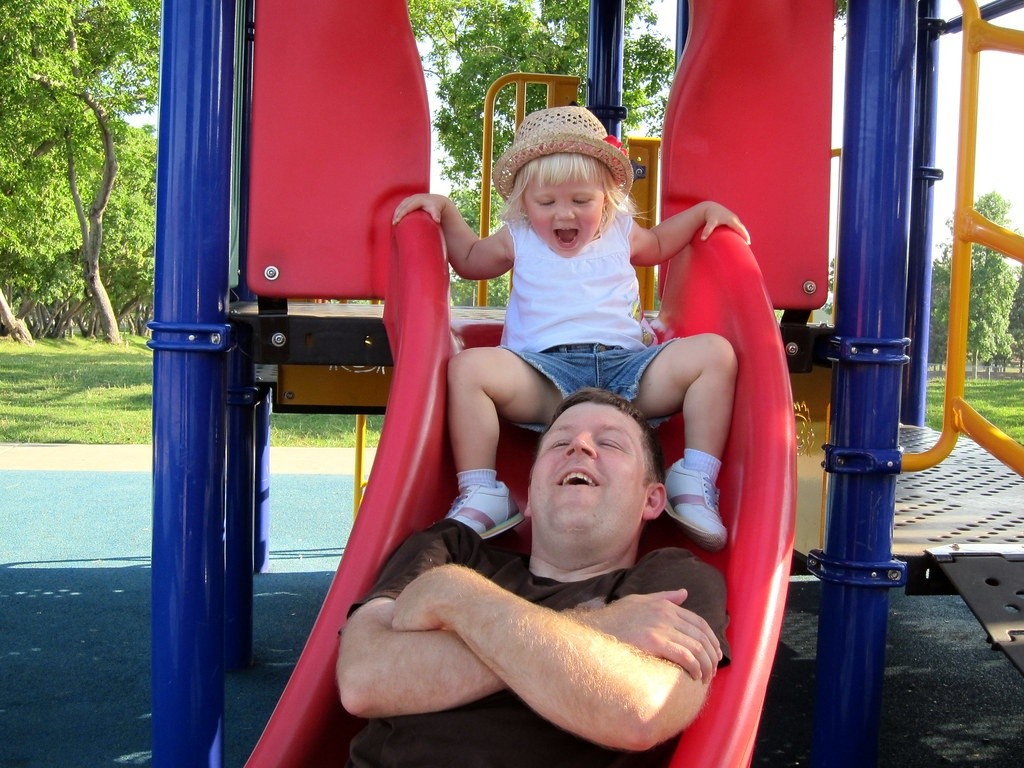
[663,457,728,551]
[443,480,525,539]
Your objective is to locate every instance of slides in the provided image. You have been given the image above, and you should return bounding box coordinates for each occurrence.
[241,200,803,768]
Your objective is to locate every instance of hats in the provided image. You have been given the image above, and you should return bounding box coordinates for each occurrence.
[493,106,634,206]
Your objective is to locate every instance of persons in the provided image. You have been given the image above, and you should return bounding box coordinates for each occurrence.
[319,388,730,768]
[392,105,750,550]
